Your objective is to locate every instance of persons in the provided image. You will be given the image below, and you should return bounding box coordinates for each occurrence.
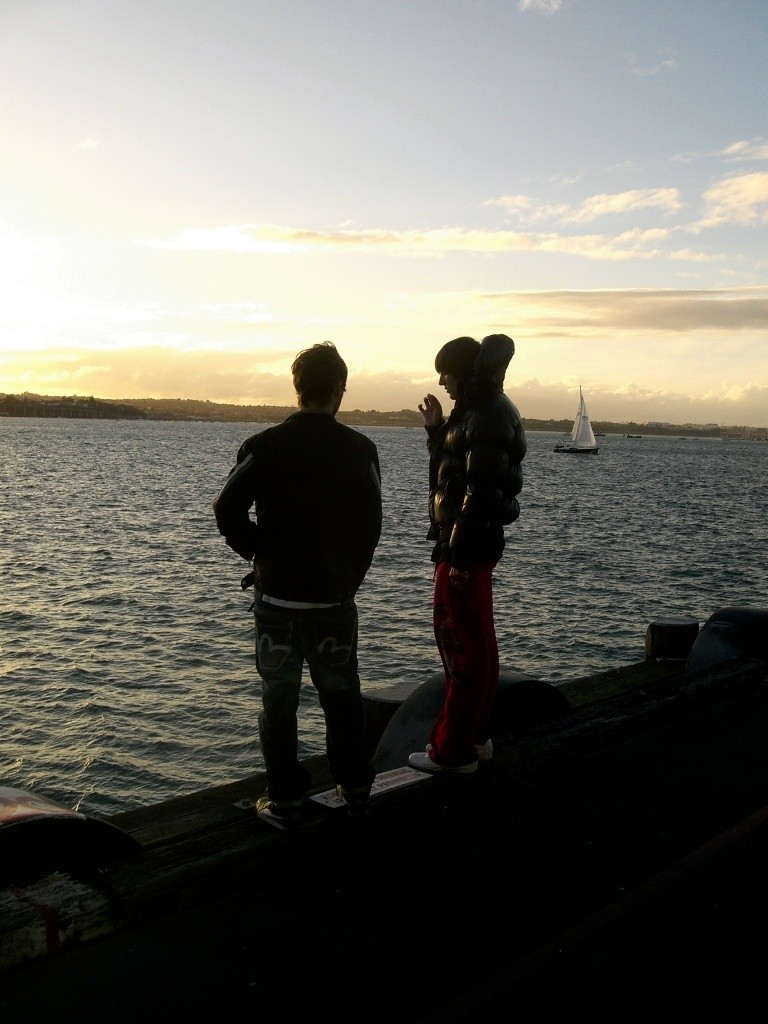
[408,334,529,775]
[213,344,383,834]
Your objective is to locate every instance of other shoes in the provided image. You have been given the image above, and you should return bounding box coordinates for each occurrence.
[334,783,370,812]
[408,751,481,775]
[257,798,296,833]
[427,739,495,760]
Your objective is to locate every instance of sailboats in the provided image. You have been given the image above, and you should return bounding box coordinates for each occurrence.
[552,382,601,455]
